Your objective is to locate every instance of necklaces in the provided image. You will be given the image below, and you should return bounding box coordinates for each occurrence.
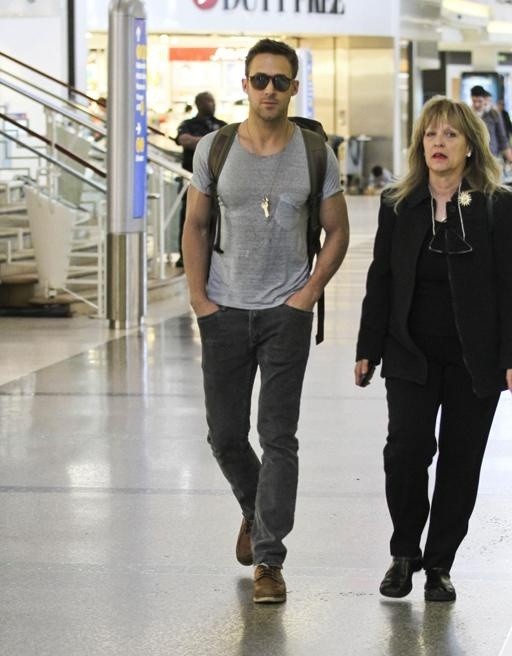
[242,119,293,221]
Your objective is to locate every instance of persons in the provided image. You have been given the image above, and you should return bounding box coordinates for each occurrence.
[182,39,349,605]
[468,83,511,172]
[227,571,291,656]
[362,164,394,196]
[493,96,511,145]
[353,96,512,603]
[483,91,494,107]
[176,90,228,268]
[382,595,472,656]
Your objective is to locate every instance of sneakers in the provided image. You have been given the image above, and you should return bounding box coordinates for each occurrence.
[235,515,256,567]
[252,566,286,603]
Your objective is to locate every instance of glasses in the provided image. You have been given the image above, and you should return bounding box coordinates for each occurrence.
[248,72,293,92]
[428,218,473,256]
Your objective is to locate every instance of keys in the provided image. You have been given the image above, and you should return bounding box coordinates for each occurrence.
[259,201,272,219]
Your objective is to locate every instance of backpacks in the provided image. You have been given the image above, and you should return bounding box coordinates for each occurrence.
[211,117,331,271]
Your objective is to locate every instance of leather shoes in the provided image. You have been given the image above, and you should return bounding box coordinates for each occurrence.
[424,569,456,601]
[379,548,423,597]
